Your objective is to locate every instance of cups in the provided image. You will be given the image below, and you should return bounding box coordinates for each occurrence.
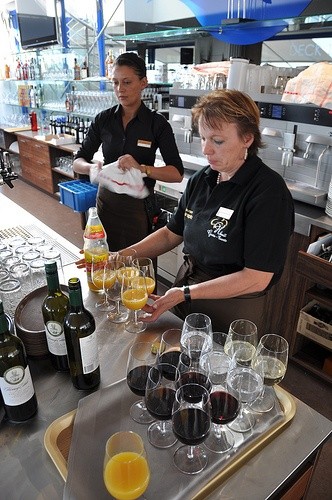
[275,76,294,94]
[0,112,30,127]
[174,73,226,90]
[102,430,150,500]
[0,237,65,318]
[43,70,73,81]
[59,156,74,175]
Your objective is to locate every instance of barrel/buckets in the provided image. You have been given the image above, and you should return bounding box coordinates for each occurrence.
[227,58,272,94]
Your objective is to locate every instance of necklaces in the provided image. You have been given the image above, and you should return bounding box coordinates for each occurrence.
[217,173,221,184]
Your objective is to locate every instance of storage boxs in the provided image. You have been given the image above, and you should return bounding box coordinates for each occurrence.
[59,179,98,211]
[297,300,332,349]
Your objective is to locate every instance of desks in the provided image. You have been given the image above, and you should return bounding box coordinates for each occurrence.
[0,193,332,500]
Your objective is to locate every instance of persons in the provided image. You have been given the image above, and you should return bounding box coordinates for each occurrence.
[76,89,295,365]
[73,52,185,295]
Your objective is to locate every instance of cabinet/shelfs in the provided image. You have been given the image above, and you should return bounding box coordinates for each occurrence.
[262,225,332,389]
[0,79,113,118]
[15,129,83,201]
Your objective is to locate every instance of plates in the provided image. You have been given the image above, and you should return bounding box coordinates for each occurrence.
[325,179,332,217]
[4,284,70,356]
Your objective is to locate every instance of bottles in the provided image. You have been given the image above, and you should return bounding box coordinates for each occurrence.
[4,64,9,78]
[106,49,123,80]
[41,261,71,371]
[29,83,43,109]
[41,110,93,144]
[29,110,37,131]
[83,207,109,292]
[66,85,78,112]
[0,299,39,427]
[63,277,101,391]
[142,87,169,109]
[72,58,80,80]
[80,61,88,79]
[29,58,41,80]
[63,58,68,70]
[16,61,28,80]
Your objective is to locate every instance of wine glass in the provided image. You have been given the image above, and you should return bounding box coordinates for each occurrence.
[67,91,116,115]
[125,313,288,475]
[91,248,155,333]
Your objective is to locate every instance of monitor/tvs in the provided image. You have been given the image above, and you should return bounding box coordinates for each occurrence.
[14,0,58,49]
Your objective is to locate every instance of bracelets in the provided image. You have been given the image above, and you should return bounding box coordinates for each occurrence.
[183,286,191,301]
